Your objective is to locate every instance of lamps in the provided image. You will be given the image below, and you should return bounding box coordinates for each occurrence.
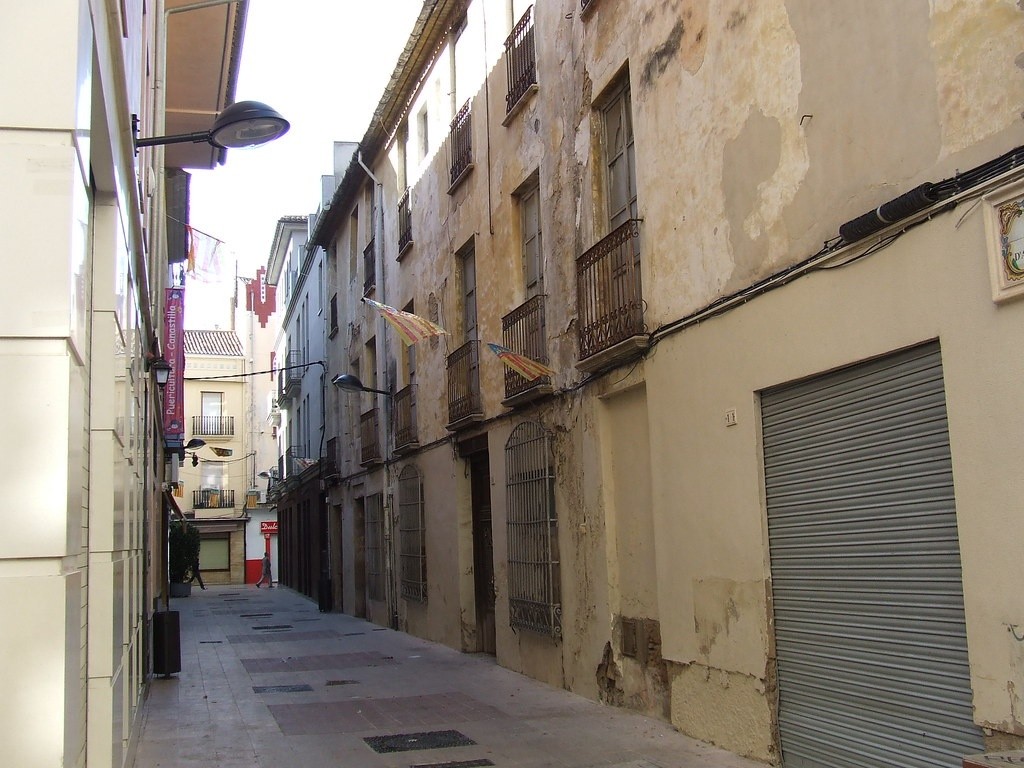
[163,439,206,457]
[257,472,278,481]
[333,374,391,396]
[148,354,173,390]
[132,100,290,157]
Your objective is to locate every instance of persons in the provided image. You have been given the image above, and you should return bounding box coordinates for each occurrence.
[256,553,274,588]
[190,558,207,589]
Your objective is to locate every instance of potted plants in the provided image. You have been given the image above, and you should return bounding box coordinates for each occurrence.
[168,520,206,597]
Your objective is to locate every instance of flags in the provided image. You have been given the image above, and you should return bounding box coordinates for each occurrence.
[487,343,553,381]
[364,297,451,346]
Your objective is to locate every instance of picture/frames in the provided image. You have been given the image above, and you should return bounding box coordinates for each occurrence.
[983,177,1024,303]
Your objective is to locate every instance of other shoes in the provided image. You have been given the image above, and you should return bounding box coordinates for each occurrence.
[201,587,208,591]
[256,584,260,588]
[269,586,275,589]
